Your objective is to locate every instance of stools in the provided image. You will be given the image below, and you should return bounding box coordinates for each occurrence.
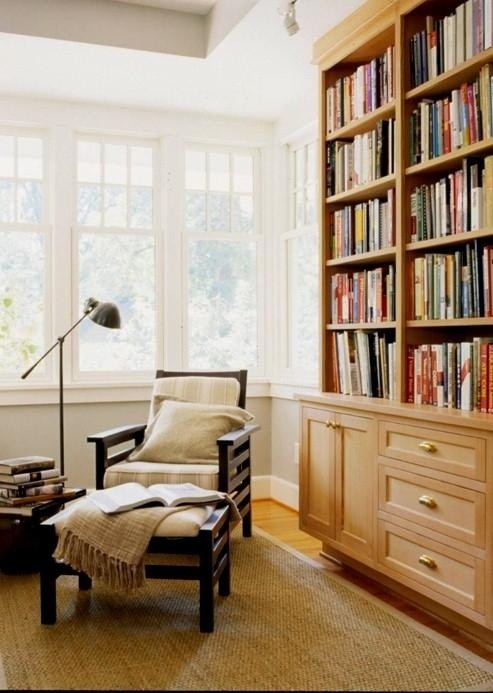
[38,495,231,634]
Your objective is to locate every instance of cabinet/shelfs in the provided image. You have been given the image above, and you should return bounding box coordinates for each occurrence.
[295,0,493,659]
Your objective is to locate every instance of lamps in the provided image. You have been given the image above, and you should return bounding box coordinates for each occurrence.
[19,297,121,487]
[285,0,299,36]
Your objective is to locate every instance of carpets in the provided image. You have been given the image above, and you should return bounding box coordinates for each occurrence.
[0,525,493,692]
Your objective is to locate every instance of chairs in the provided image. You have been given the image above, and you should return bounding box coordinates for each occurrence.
[86,370,260,560]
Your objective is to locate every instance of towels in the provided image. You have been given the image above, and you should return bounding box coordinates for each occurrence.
[51,487,243,592]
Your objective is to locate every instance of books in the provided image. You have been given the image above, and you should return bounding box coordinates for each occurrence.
[327,264,396,401]
[326,45,394,131]
[406,1,493,243]
[326,118,396,260]
[406,247,493,413]
[88,482,226,515]
[0,456,87,517]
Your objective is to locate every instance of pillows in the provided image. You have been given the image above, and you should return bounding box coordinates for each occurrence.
[125,393,254,464]
